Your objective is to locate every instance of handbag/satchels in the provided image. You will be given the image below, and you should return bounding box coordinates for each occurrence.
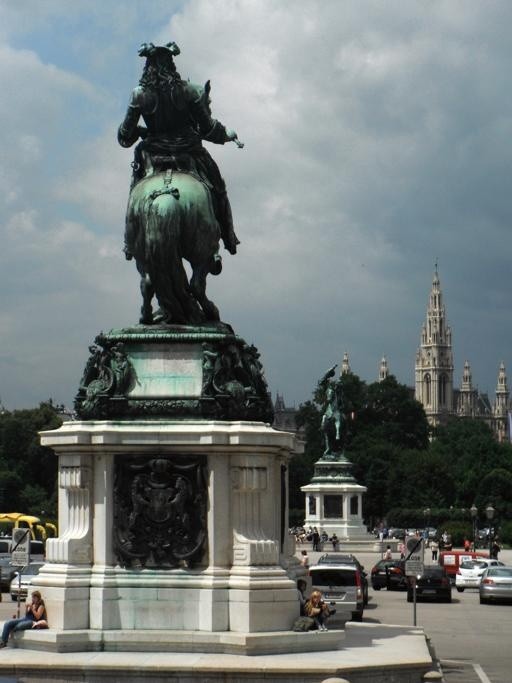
[32,619,48,629]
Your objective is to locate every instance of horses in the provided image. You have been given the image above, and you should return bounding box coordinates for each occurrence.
[319,383,348,454]
[122,77,221,324]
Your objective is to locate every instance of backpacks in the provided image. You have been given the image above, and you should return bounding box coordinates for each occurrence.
[293,616,314,632]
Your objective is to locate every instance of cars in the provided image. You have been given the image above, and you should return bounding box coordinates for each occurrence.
[439,534,453,550]
[475,527,499,547]
[371,551,512,605]
[373,527,436,547]
[308,553,368,620]
[0,512,57,600]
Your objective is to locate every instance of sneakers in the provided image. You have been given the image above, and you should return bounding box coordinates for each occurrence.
[318,624,327,631]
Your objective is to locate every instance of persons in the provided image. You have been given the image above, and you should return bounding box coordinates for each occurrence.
[117,41,240,255]
[318,363,344,454]
[290,521,500,631]
[76,341,131,395]
[202,342,266,393]
[0,591,45,648]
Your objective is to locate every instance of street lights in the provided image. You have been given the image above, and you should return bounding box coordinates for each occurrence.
[380,533,384,560]
[470,503,478,552]
[486,504,495,556]
